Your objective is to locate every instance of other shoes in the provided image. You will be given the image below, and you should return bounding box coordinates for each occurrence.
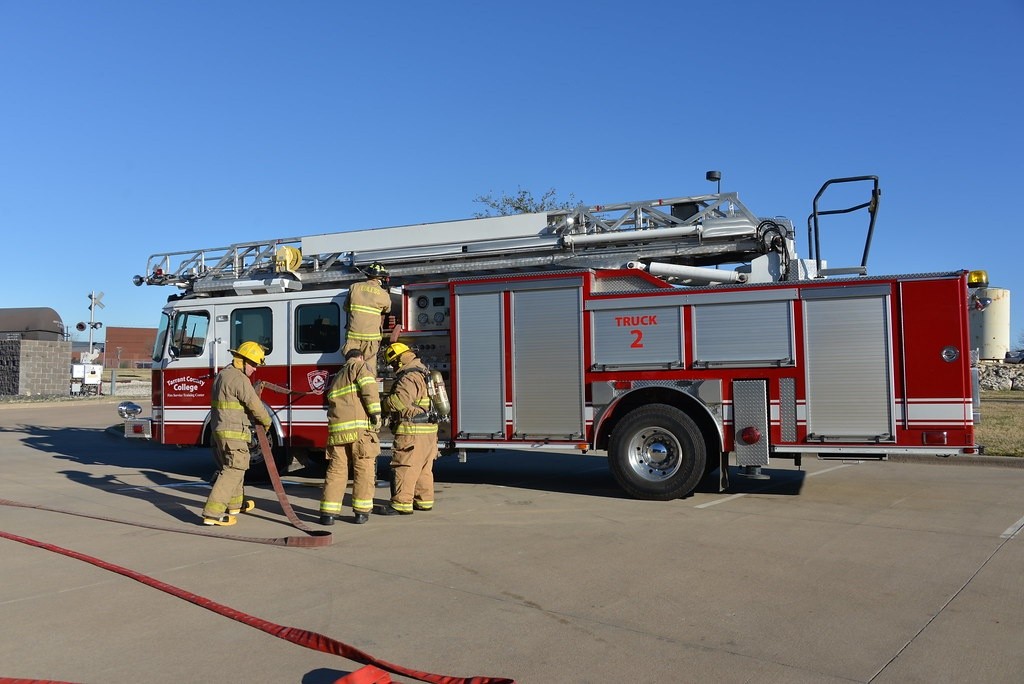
[355,513,368,523]
[373,504,403,515]
[204,514,237,526]
[319,515,334,525]
[228,499,255,515]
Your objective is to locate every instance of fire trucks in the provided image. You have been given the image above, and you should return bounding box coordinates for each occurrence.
[117,171,989,501]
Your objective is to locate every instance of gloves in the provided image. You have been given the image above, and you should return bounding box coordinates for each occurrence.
[264,425,269,434]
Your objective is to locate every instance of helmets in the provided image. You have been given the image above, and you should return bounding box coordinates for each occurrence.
[227,341,266,367]
[365,261,390,279]
[385,343,410,366]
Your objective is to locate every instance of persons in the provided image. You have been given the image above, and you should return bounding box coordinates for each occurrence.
[381,344,439,515]
[203,342,272,526]
[318,349,382,525]
[341,264,392,377]
